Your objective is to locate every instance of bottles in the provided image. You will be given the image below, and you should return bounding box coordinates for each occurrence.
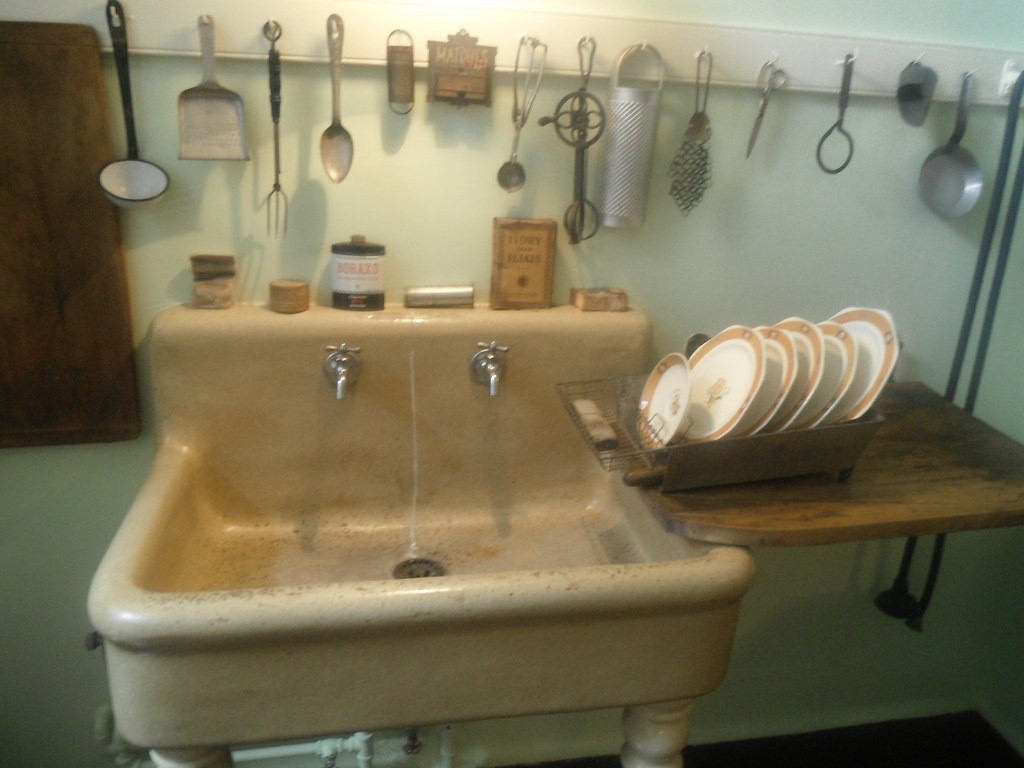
[330,234,387,312]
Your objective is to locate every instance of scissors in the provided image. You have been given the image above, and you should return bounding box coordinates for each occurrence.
[746,61,788,158]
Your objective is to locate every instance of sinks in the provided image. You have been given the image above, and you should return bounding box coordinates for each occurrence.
[84,407,755,748]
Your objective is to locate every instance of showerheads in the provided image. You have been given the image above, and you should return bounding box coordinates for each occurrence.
[326,345,361,401]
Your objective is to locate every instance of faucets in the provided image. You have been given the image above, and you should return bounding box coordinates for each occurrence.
[473,340,511,397]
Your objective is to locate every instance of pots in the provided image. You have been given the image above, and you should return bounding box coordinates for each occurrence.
[917,70,985,220]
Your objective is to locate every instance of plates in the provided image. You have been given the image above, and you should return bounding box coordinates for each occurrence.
[754,316,827,437]
[812,305,900,428]
[670,324,766,444]
[786,321,860,432]
[722,325,798,441]
[635,352,693,450]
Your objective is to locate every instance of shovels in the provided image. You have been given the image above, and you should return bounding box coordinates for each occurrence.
[176,12,251,162]
